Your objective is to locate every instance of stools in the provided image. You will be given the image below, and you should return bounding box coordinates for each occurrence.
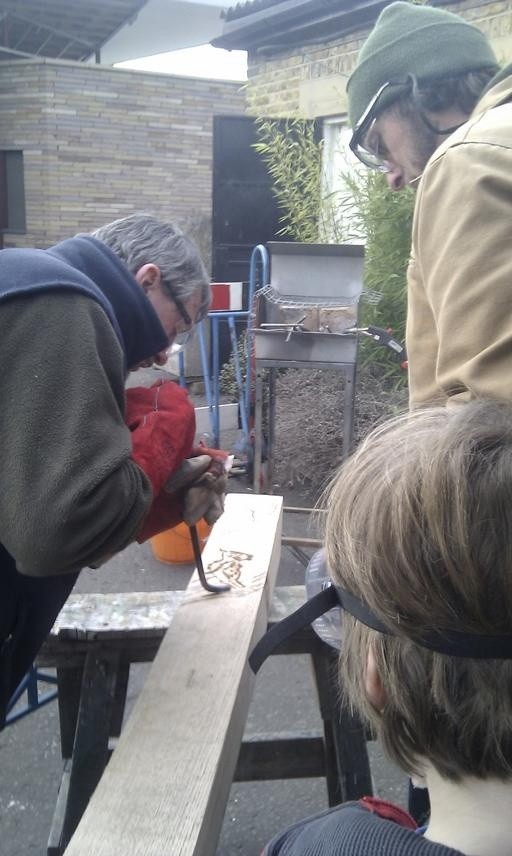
[36,582,379,856]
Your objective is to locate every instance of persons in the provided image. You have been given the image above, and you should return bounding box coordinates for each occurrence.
[259,395,512,856]
[0,209,225,723]
[342,0,510,413]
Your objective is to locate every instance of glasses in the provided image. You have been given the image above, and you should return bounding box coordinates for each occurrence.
[348,78,408,175]
[159,275,195,358]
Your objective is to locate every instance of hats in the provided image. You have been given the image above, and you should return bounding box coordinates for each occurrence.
[346,1,500,126]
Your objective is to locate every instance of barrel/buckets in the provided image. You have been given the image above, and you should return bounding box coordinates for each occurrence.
[151,516,212,564]
